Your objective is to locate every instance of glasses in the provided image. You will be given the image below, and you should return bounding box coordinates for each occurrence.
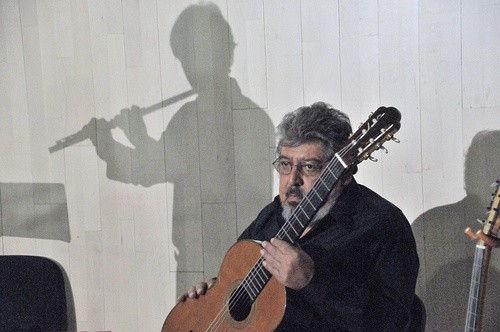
[273,156,332,176]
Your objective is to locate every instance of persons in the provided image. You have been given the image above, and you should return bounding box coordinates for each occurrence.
[174,103,428,332]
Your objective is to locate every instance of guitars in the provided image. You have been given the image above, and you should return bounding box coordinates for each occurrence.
[465,179,500,331]
[163,105,402,332]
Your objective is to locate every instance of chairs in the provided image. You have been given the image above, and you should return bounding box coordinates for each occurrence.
[411,293,428,332]
[0,254,68,332]
